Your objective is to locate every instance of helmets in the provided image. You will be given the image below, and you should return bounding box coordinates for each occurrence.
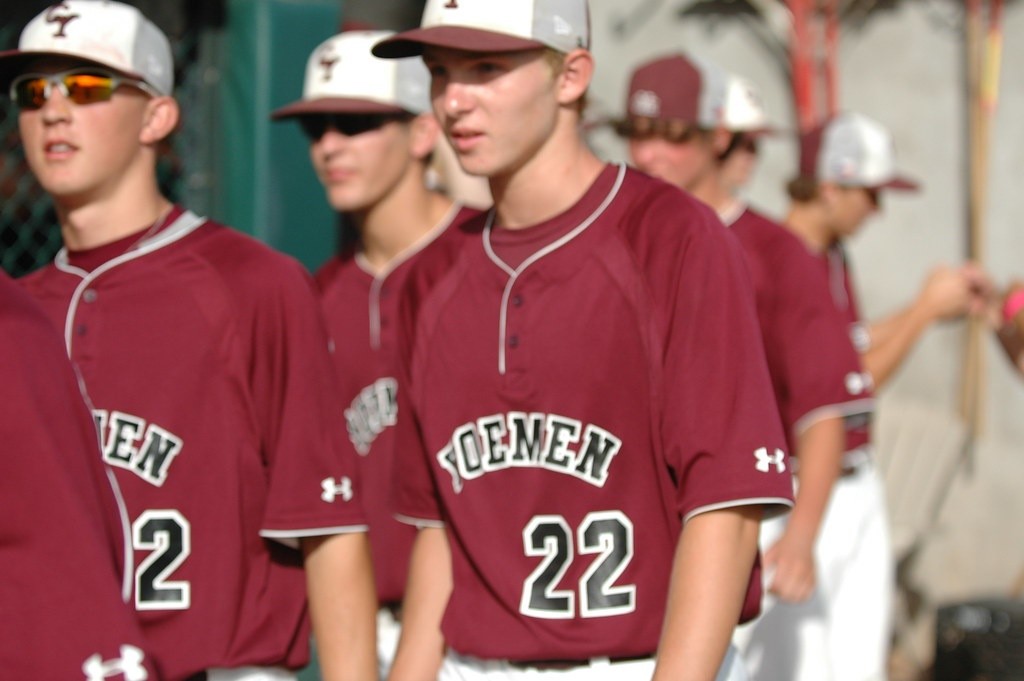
[626,50,729,129]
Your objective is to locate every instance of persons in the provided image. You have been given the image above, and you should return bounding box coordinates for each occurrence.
[607,47,1023,681]
[372,0,797,681]
[265,29,489,681]
[0,0,379,681]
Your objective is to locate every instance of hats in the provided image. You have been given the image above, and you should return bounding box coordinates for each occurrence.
[801,113,919,191]
[714,68,783,137]
[270,30,432,116]
[0,0,175,98]
[371,0,592,59]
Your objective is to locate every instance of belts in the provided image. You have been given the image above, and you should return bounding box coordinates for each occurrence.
[507,652,652,667]
[839,464,859,481]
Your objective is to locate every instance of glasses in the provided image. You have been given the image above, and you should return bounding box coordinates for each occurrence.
[624,114,692,143]
[9,67,158,110]
[299,113,416,140]
